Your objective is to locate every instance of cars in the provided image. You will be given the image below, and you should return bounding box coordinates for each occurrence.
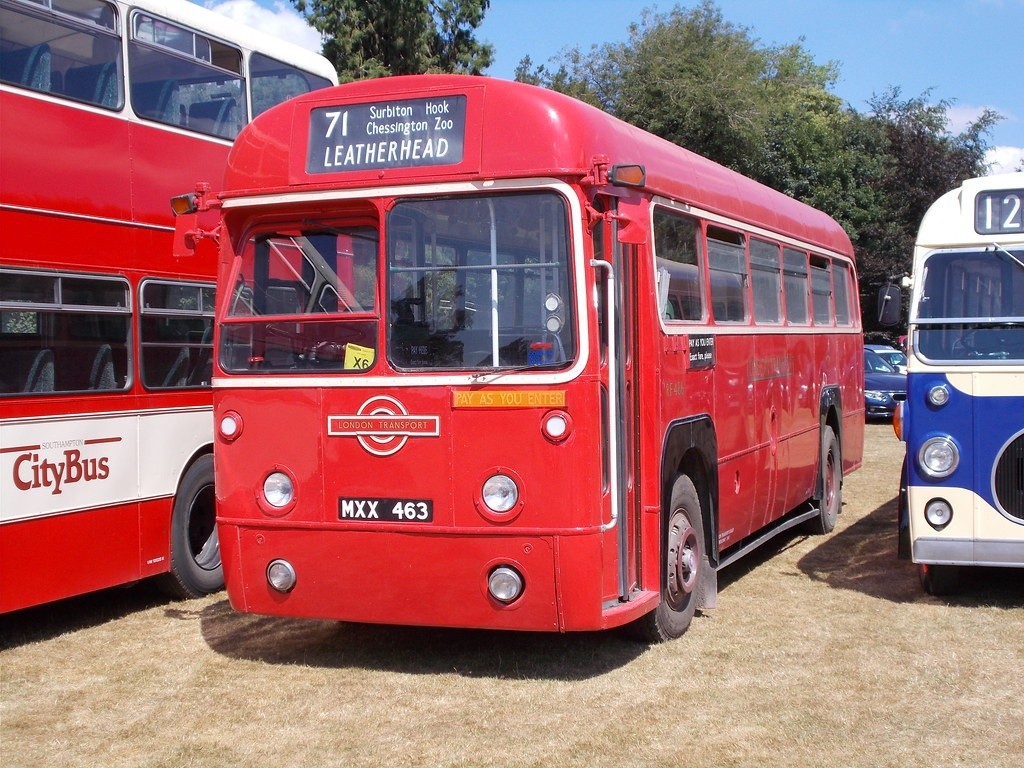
[863,344,907,423]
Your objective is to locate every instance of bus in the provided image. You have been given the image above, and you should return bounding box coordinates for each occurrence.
[877,172,1024,597]
[0,0,352,616]
[171,74,865,643]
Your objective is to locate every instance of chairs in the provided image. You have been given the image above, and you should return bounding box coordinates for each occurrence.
[22,330,217,392]
[973,327,1024,352]
[434,330,521,367]
[0,41,236,136]
